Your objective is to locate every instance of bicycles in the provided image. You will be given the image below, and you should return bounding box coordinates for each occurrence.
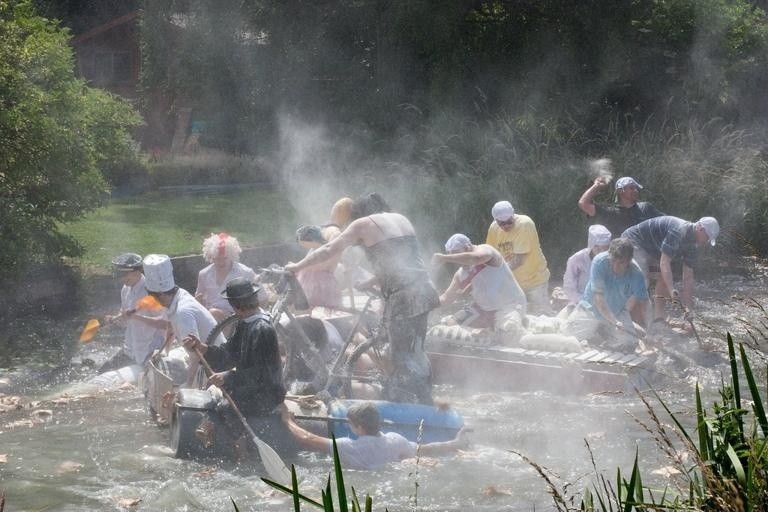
[205,267,435,401]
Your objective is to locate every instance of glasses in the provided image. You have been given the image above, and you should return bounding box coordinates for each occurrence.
[498,220,512,226]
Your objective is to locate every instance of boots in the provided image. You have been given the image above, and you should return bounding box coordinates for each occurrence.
[227,433,254,477]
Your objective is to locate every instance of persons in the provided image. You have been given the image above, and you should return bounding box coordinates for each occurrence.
[182,275,288,461]
[142,255,235,391]
[485,200,551,311]
[431,233,526,345]
[194,232,270,343]
[281,402,470,468]
[284,191,442,405]
[559,238,650,354]
[363,319,433,408]
[334,245,377,293]
[295,197,356,317]
[275,316,346,402]
[621,216,720,327]
[97,253,169,377]
[556,224,612,321]
[577,175,668,293]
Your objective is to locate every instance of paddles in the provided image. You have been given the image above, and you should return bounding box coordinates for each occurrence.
[79,295,162,343]
[194,347,296,487]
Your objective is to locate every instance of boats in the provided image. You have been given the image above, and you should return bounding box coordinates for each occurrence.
[419,280,697,396]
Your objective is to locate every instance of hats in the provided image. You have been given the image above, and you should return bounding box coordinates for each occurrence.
[700,216,720,246]
[219,277,260,300]
[492,200,514,221]
[143,253,175,292]
[111,252,143,272]
[445,233,470,252]
[587,224,612,255]
[615,177,643,192]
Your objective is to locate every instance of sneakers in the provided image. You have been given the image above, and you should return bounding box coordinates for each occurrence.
[650,320,672,335]
